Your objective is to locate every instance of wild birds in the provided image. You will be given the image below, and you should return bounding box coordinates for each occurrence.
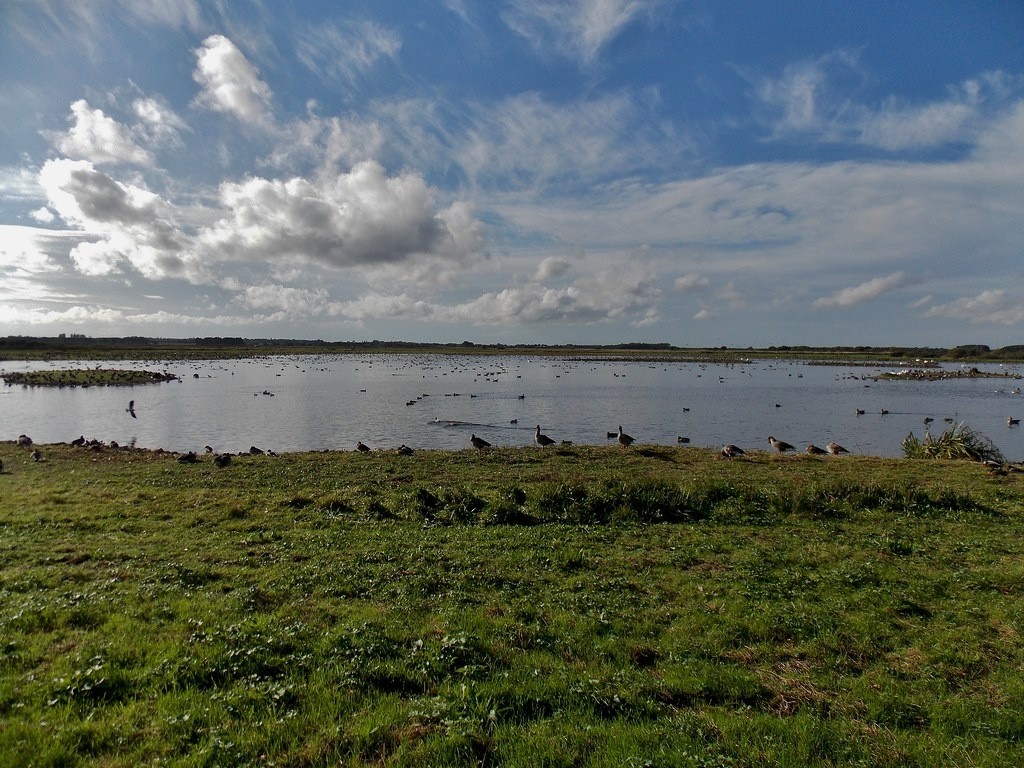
[2,351,1024,455]
